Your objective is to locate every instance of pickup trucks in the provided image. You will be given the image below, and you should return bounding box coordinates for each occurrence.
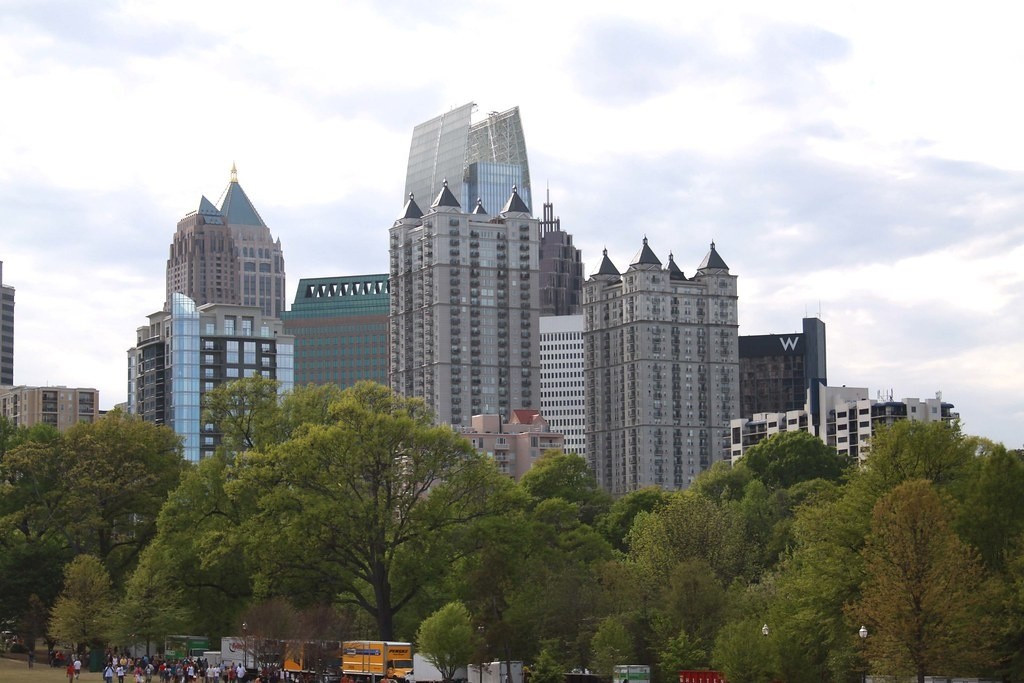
[283,639,343,683]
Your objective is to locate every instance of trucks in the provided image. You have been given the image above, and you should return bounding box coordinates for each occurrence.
[203,651,221,667]
[164,634,211,665]
[342,641,414,683]
[219,636,282,676]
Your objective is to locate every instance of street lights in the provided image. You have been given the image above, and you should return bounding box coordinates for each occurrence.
[858,625,868,683]
[241,621,249,671]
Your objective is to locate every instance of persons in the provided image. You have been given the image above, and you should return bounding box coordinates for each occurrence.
[381,677,389,683]
[523,667,533,683]
[28,649,34,668]
[340,675,354,683]
[49,651,314,683]
